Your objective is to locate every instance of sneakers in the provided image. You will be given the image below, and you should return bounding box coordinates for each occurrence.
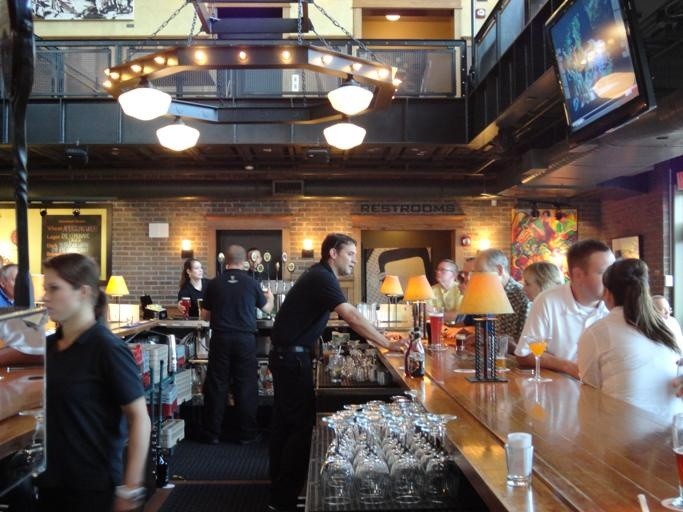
[239,431,264,444]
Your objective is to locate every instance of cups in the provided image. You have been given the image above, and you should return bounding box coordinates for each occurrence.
[260,279,294,295]
[321,338,392,385]
[425,320,432,345]
[456,334,465,352]
[429,307,444,346]
[669,412,683,508]
[489,336,508,369]
[676,358,683,398]
[504,433,533,487]
[182,298,192,320]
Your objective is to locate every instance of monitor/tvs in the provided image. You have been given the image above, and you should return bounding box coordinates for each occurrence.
[545,0,657,144]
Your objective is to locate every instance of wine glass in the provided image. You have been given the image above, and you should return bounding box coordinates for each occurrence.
[523,335,553,382]
[320,389,460,504]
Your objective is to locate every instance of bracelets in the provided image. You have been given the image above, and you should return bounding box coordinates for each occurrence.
[114,486,146,501]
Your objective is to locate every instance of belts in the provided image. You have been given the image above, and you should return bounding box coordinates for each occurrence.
[271,344,303,352]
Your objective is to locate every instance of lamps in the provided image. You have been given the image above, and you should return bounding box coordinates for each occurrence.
[379,274,403,330]
[404,275,437,344]
[181,238,194,258]
[94,0,401,158]
[459,270,514,383]
[299,237,316,259]
[103,274,129,326]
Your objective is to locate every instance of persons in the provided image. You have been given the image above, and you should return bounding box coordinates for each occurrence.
[651,295,672,317]
[38,253,151,512]
[201,245,274,445]
[178,259,211,318]
[432,248,565,351]
[578,259,683,417]
[0,263,19,311]
[514,240,618,379]
[268,234,411,512]
[246,247,264,283]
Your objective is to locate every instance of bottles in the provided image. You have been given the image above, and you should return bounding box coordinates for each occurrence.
[404,327,425,377]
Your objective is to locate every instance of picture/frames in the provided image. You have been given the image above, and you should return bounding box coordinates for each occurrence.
[609,234,642,259]
